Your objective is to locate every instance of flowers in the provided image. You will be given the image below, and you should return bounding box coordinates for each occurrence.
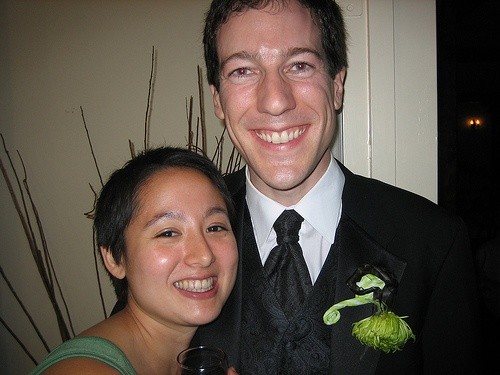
[351,307,417,356]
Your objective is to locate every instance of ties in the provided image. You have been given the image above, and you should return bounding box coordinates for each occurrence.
[264,209,313,326]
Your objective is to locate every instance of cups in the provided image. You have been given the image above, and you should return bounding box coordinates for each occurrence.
[176,345,229,375]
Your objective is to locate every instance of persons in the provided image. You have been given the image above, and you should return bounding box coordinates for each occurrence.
[116,1,495,375]
[22,147,242,375]
